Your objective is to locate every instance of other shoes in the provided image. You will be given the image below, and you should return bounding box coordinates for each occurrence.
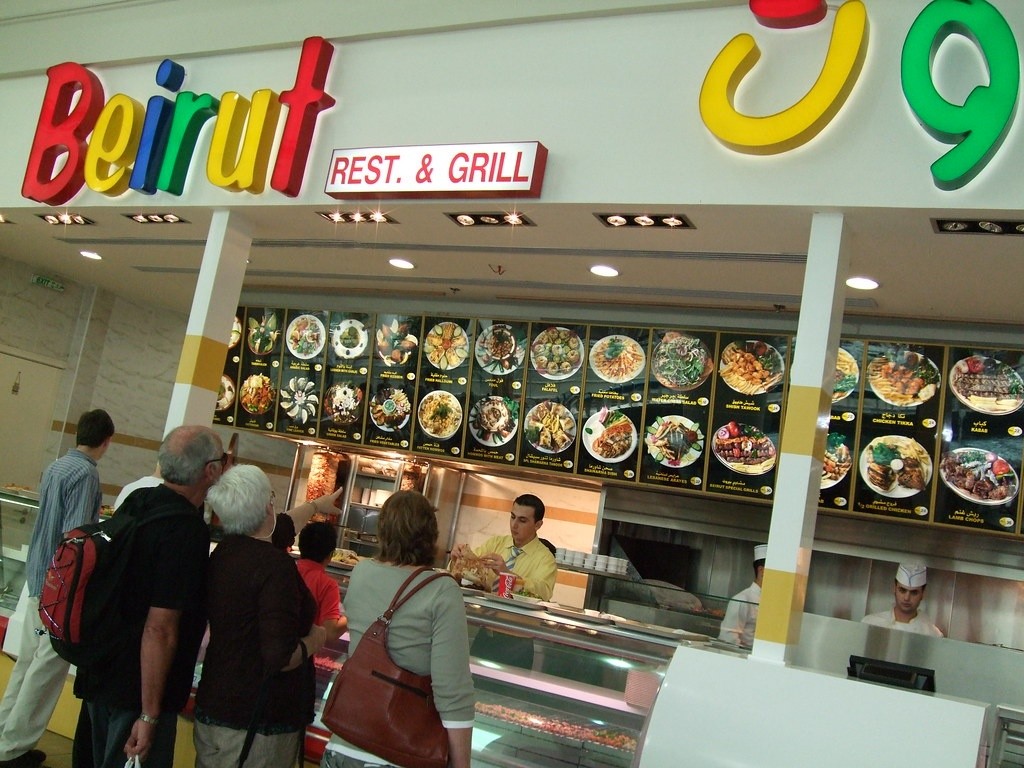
[27,750,47,762]
[0,753,51,768]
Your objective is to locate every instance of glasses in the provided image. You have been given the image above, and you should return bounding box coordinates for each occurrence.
[206,453,228,466]
[270,491,275,504]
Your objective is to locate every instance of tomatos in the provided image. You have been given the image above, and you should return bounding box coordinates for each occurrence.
[729,422,740,437]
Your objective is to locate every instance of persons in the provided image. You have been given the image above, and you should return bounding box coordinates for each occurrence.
[294,520,349,648]
[862,563,946,640]
[442,494,559,700]
[317,492,476,768]
[113,461,163,514]
[70,424,226,768]
[0,408,115,768]
[191,465,344,768]
[717,544,767,650]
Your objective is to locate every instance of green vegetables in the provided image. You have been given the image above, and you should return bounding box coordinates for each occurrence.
[503,397,519,420]
[914,363,939,384]
[662,339,703,386]
[827,433,846,452]
[835,376,856,391]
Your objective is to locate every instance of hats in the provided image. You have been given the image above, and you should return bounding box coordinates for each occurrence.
[754,545,769,560]
[896,564,928,587]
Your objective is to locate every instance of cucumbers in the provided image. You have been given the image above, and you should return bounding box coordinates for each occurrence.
[655,452,664,463]
[454,326,462,337]
[440,356,448,372]
[435,325,443,334]
[697,434,705,440]
[656,416,664,426]
[456,349,469,358]
[691,422,699,431]
[646,426,657,435]
[424,346,435,352]
[691,442,702,451]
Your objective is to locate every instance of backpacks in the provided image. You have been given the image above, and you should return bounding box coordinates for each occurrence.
[38,502,190,665]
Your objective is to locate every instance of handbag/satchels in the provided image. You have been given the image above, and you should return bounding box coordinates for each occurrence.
[125,754,141,768]
[321,567,457,768]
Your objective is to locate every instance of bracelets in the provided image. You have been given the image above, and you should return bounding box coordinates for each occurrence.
[307,500,318,513]
[139,713,161,725]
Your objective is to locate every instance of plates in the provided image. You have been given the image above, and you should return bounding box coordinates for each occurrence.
[370,388,410,432]
[868,351,941,406]
[286,314,326,359]
[859,435,932,498]
[240,383,275,414]
[331,319,368,359]
[324,382,362,425]
[210,373,235,411]
[248,332,277,355]
[228,316,242,349]
[790,347,859,403]
[939,448,1019,505]
[418,315,785,475]
[820,434,847,489]
[949,356,1024,415]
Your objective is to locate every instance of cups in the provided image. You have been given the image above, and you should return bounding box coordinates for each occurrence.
[498,571,517,600]
[555,547,628,575]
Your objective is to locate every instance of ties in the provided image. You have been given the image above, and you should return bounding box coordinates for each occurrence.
[492,547,522,592]
